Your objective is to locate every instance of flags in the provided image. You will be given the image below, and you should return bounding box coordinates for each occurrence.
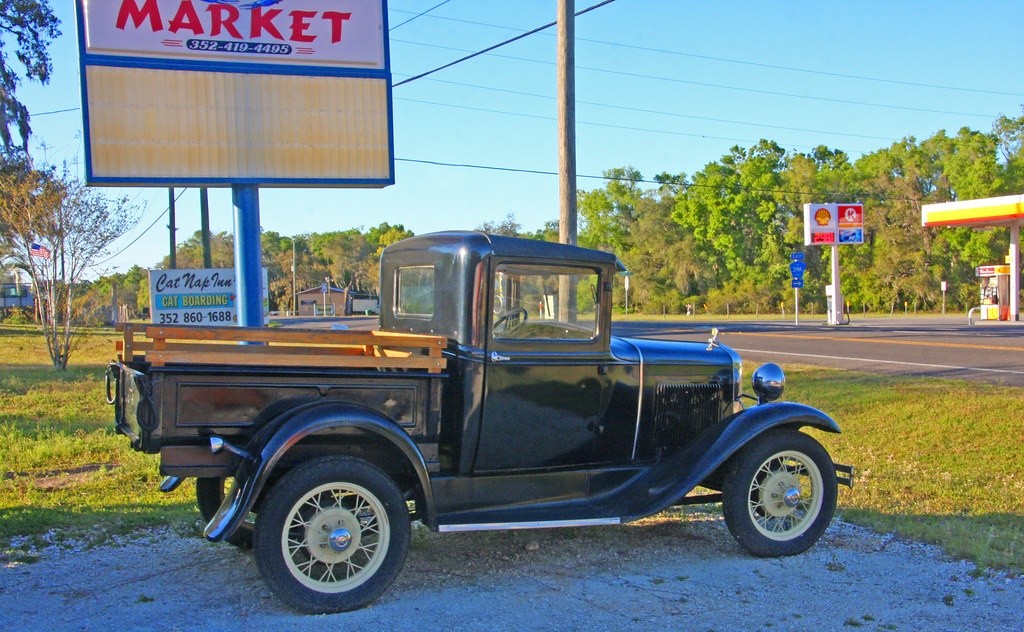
[29,242,51,259]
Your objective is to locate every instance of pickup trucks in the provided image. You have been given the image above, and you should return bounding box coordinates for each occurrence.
[108,228,863,612]
